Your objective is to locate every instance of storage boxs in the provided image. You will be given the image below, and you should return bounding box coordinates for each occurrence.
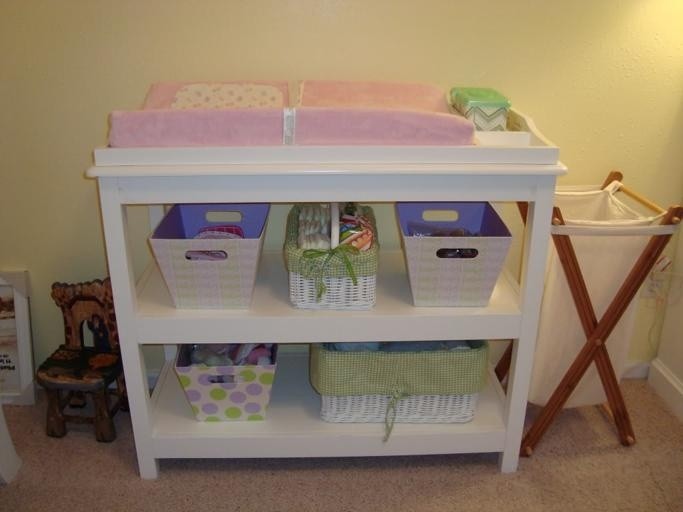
[148,203,269,311]
[174,343,276,424]
[451,87,510,131]
[394,201,511,308]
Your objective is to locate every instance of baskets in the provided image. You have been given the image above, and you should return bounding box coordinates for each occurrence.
[308,341,490,425]
[282,202,380,311]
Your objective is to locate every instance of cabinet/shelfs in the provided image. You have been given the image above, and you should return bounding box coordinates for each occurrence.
[86,161,568,481]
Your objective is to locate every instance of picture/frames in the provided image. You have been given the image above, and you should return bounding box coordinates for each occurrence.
[0,270,36,406]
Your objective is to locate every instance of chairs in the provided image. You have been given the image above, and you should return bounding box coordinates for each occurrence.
[36,277,129,442]
